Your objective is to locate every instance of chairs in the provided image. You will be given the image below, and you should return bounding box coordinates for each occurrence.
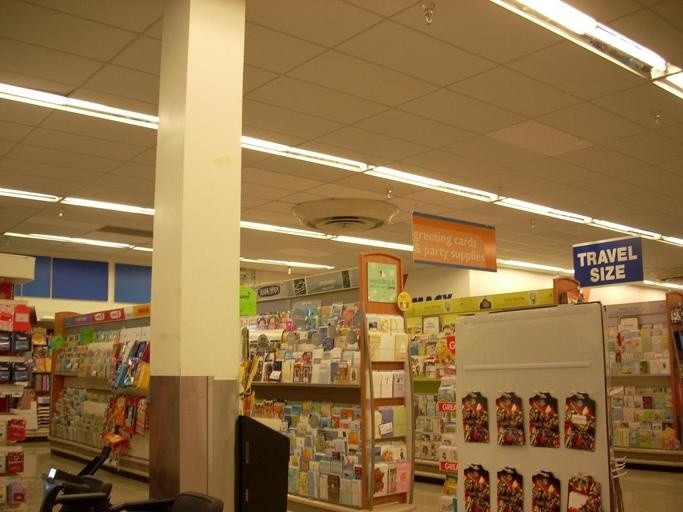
[39,444,227,512]
[233,412,294,512]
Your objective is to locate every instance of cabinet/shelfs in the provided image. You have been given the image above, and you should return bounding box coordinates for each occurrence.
[0,249,36,510]
[451,300,625,512]
[32,318,54,439]
[402,276,587,485]
[584,291,683,471]
[47,249,415,512]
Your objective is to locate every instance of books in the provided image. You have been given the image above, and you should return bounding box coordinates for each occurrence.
[242,299,361,509]
[367,314,456,509]
[605,316,679,449]
